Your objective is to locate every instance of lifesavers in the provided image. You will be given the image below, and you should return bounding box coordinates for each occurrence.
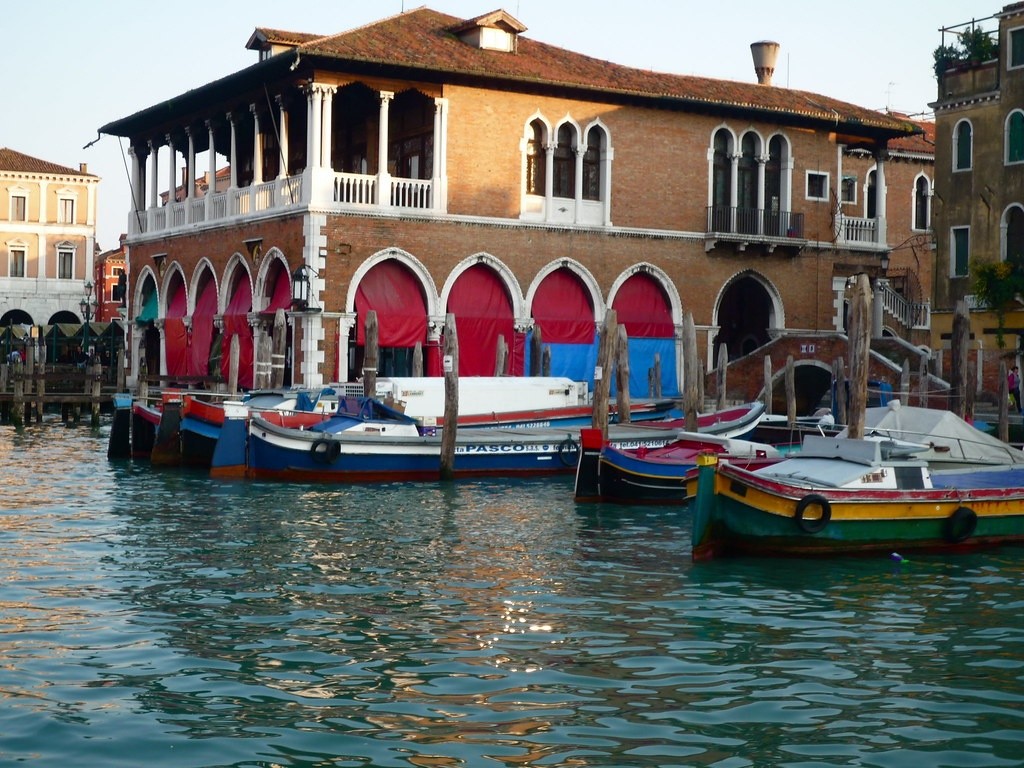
[795,495,831,533]
[945,507,978,543]
[311,438,341,464]
[559,439,580,466]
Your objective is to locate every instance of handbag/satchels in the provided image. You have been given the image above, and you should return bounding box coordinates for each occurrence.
[1008,394,1016,410]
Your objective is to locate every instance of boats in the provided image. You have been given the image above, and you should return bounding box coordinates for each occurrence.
[106,377,995,504]
[681,399,1024,562]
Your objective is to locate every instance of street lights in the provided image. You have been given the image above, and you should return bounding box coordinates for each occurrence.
[78,281,101,362]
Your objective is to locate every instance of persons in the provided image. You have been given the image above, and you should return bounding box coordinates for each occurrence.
[1008,366,1022,413]
[11,348,26,362]
[77,346,111,366]
